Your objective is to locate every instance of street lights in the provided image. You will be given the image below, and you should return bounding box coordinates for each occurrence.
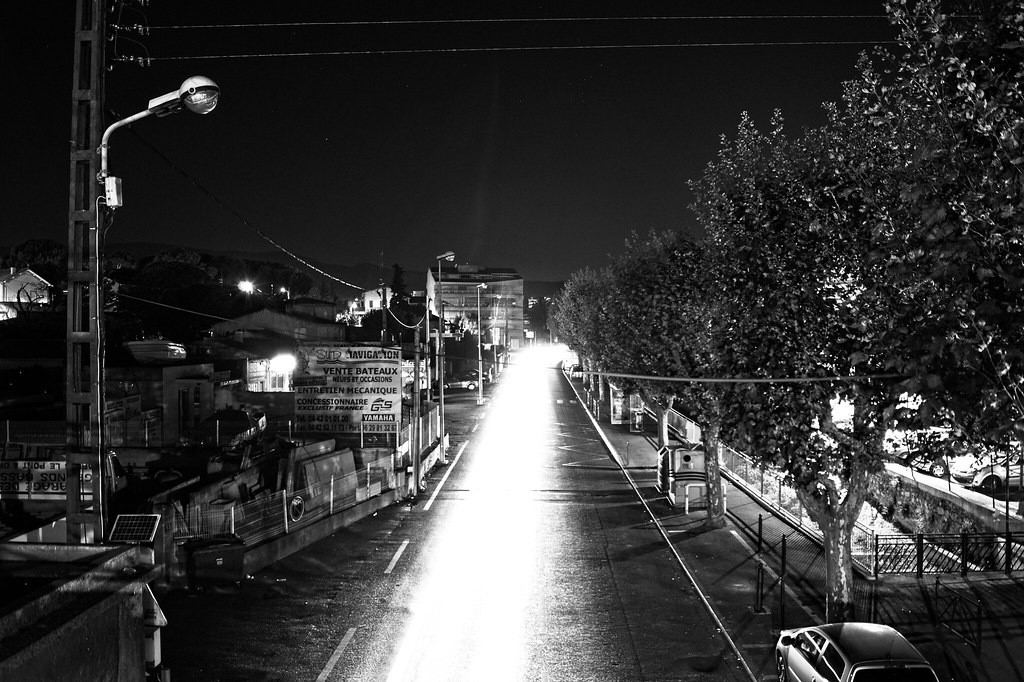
[493,295,503,380]
[475,282,490,406]
[504,299,516,359]
[437,250,456,466]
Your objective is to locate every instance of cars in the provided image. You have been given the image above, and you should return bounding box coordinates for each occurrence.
[775,622,941,682]
[432,369,488,391]
[884,420,1024,496]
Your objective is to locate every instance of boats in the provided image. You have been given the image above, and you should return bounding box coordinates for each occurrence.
[123,328,188,365]
